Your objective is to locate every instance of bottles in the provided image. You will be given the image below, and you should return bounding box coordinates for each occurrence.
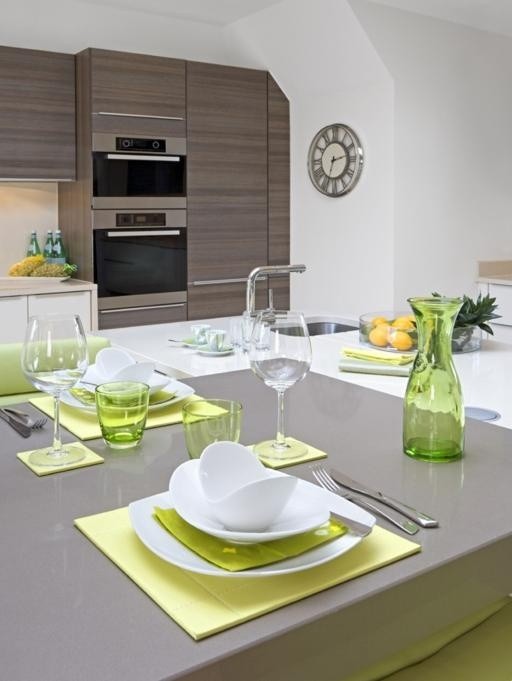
[402,297,464,461]
[26,229,65,259]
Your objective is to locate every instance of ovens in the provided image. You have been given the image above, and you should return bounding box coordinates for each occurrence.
[90,151,188,313]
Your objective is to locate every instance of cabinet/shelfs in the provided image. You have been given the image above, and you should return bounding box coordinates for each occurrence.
[78,49,186,138]
[188,62,268,321]
[0,45,78,184]
[476,280,512,323]
[1,283,98,343]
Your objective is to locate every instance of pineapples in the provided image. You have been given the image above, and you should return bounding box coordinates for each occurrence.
[35,263,71,279]
[9,255,41,277]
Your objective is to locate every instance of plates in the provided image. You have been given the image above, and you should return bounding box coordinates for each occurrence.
[181,340,208,347]
[167,454,328,540]
[196,345,235,355]
[81,361,168,398]
[58,368,193,413]
[117,467,366,574]
[358,311,480,354]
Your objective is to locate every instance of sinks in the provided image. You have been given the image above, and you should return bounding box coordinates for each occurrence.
[270,321,360,337]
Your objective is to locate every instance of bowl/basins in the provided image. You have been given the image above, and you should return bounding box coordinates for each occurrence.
[95,348,139,384]
[199,440,288,530]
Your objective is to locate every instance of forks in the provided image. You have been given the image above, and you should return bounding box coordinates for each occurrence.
[8,406,47,429]
[309,464,419,534]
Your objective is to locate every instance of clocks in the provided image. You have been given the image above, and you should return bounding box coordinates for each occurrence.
[307,123,363,198]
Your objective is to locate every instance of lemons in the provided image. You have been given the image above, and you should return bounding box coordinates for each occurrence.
[365,314,415,350]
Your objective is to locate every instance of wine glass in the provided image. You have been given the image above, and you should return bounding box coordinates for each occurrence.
[249,310,313,460]
[20,315,98,465]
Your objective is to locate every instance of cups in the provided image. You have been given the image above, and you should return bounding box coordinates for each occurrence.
[189,325,208,342]
[205,330,225,350]
[97,381,149,451]
[182,399,242,459]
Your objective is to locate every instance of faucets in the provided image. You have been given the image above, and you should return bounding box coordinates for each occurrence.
[246,265,312,319]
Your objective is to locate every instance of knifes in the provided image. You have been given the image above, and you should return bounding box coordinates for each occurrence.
[331,468,440,527]
[1,412,30,437]
